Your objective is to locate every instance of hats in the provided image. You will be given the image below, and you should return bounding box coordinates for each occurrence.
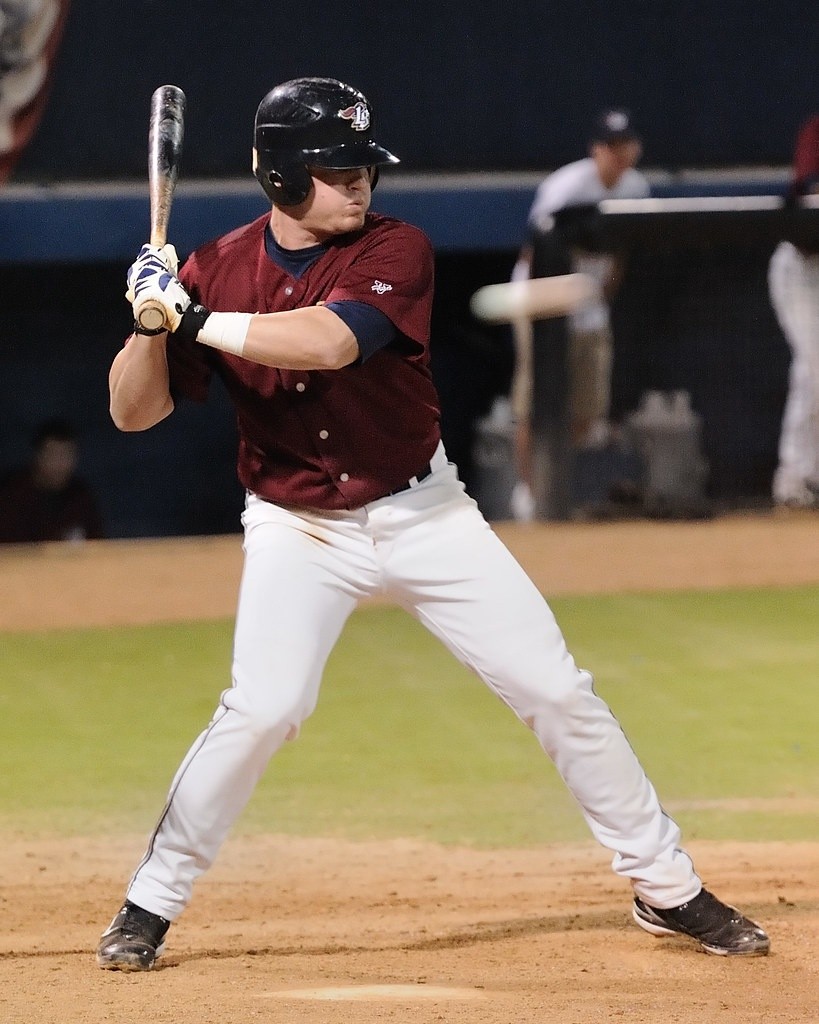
[597,109,636,143]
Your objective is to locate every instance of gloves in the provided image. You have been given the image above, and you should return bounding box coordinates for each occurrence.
[125,243,212,344]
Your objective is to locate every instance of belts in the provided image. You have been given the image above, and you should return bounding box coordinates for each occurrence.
[374,461,431,501]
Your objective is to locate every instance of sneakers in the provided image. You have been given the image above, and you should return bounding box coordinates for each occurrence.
[94,898,170,972]
[633,887,770,956]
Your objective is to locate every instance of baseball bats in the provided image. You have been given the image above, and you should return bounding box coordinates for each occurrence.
[136,82,187,332]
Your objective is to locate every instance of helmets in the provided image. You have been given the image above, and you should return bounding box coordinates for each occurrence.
[252,77,401,206]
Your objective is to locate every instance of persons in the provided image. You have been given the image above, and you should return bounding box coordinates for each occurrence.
[510,110,652,523]
[769,111,819,510]
[96,76,773,972]
